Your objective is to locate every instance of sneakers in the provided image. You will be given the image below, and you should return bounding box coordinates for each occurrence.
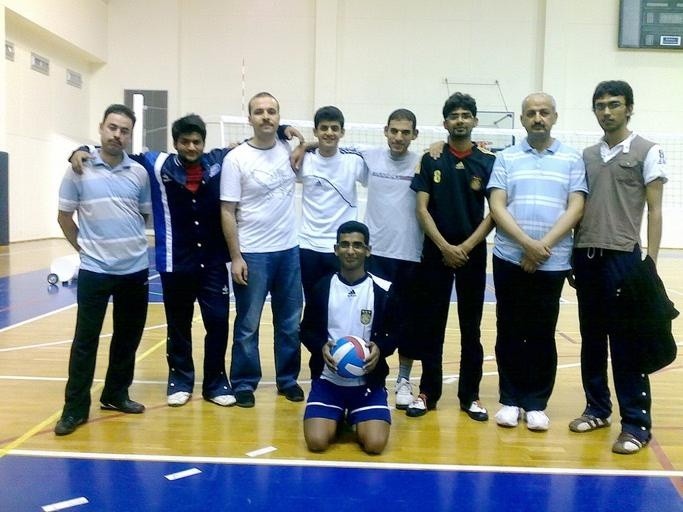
[55,382,304,436]
[395,378,651,453]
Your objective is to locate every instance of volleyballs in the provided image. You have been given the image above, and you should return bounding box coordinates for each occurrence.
[331,336,372,378]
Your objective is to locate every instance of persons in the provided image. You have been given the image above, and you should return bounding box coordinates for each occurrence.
[68,112,306,408]
[53,102,152,435]
[485,91,591,432]
[405,91,508,421]
[297,220,405,455]
[288,109,445,412]
[217,92,306,407]
[563,80,680,455]
[228,104,446,409]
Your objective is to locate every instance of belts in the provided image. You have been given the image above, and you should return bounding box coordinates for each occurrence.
[577,247,610,260]
